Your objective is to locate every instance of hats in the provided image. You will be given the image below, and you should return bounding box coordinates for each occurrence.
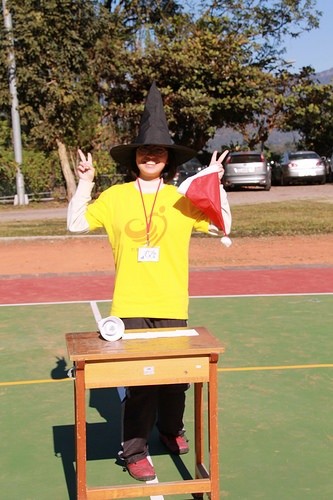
[109,77,201,167]
[175,164,231,246]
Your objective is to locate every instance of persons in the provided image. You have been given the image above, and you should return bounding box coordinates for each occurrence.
[66,79,231,482]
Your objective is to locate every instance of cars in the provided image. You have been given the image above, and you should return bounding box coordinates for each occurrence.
[222,150,273,192]
[274,150,328,187]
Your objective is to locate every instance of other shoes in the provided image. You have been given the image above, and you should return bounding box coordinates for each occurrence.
[159,433,189,455]
[126,457,156,481]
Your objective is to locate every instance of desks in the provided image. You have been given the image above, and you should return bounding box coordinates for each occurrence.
[64,324,228,500]
